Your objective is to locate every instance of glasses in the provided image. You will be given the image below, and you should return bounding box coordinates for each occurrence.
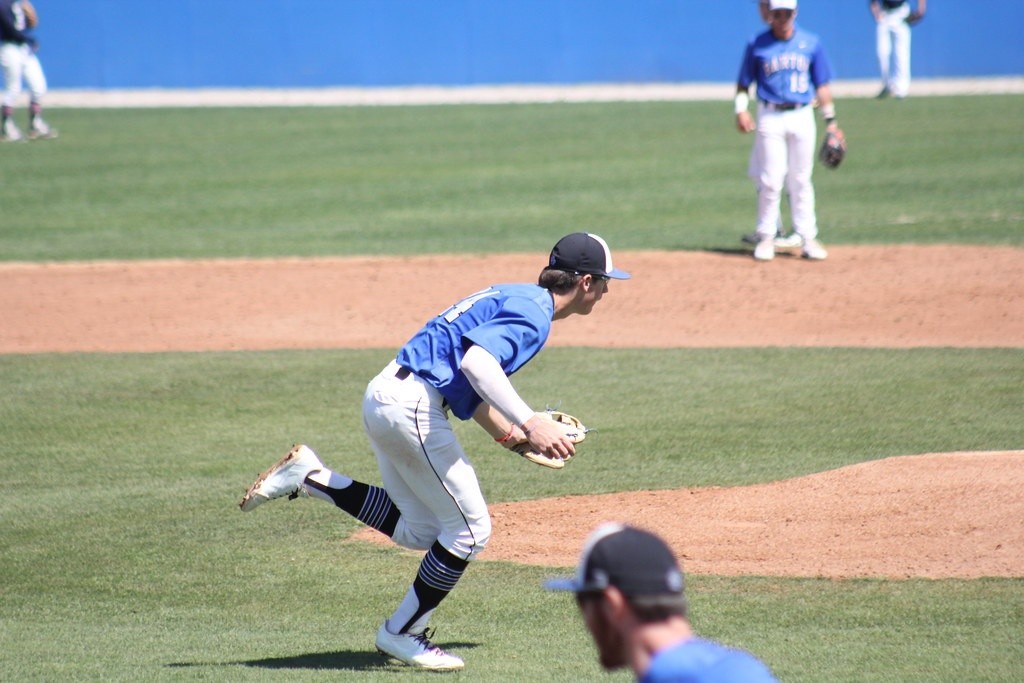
[773,11,793,18]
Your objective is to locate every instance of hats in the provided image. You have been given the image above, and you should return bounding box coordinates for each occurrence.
[546,526,681,590]
[767,0,797,11]
[550,232,632,279]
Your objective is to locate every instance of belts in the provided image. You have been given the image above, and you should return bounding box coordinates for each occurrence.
[764,101,806,110]
[395,366,448,408]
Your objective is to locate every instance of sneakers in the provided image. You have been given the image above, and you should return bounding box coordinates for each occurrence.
[374,619,465,671]
[240,444,323,511]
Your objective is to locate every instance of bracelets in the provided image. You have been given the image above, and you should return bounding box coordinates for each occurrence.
[495,422,515,444]
[523,418,543,433]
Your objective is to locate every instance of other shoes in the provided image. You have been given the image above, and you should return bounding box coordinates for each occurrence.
[29,115,59,139]
[0,115,22,141]
[801,240,828,260]
[753,238,775,260]
[876,86,891,98]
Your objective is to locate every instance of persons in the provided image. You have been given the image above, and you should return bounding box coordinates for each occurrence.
[239,232,630,672]
[0,0,59,141]
[870,0,925,99]
[734,0,848,262]
[545,520,783,683]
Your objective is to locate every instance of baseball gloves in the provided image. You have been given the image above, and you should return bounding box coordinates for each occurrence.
[510,410,587,470]
[819,127,845,171]
